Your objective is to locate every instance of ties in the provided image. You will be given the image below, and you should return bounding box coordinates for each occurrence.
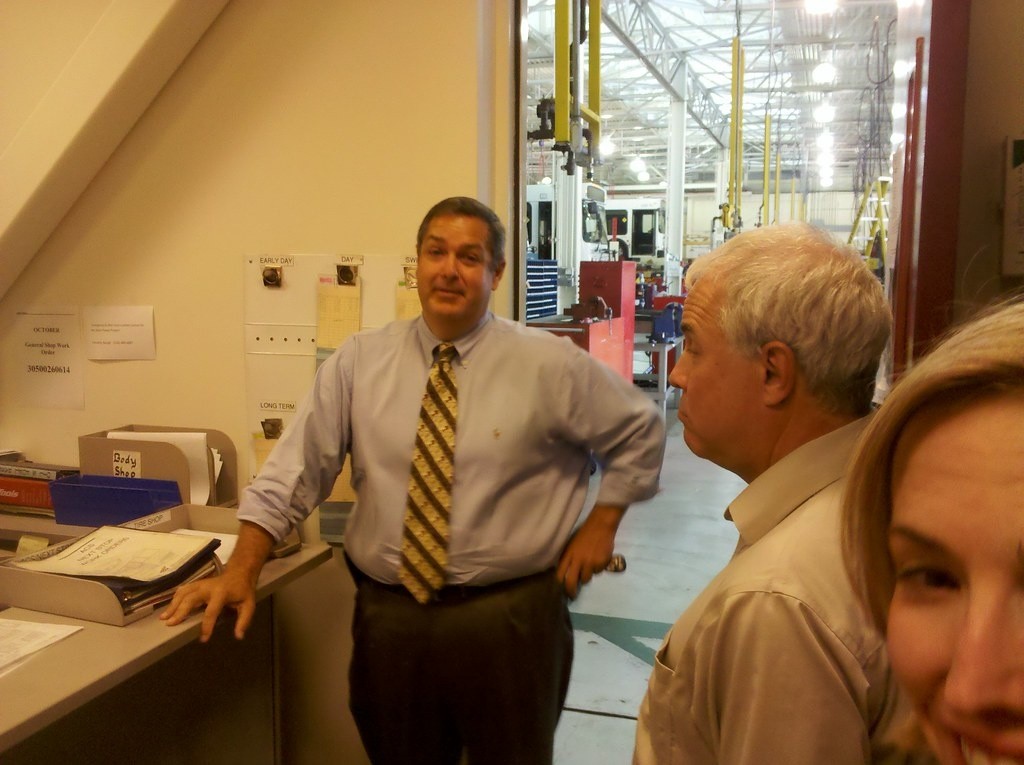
[397,343,459,607]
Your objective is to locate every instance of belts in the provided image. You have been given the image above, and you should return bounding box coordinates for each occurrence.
[353,564,557,602]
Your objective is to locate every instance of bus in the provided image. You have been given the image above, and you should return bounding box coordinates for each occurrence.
[528,181,670,282]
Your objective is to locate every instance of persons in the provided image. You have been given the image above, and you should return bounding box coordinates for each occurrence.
[833,297,1024,765]
[626,221,940,765]
[683,258,693,272]
[161,196,667,764]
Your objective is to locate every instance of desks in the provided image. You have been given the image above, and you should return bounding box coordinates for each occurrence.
[1,494,336,765]
